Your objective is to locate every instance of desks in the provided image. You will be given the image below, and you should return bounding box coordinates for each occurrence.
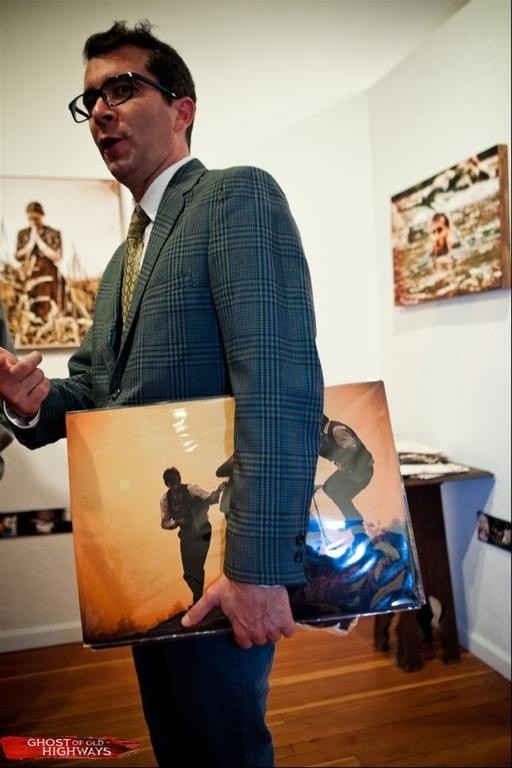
[372,452,495,673]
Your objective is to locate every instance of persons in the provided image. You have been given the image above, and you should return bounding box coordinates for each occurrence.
[317,414,376,542]
[422,211,461,259]
[214,448,237,523]
[0,20,327,768]
[14,202,63,328]
[157,466,223,611]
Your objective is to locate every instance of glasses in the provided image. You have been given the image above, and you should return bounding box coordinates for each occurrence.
[68,72,178,124]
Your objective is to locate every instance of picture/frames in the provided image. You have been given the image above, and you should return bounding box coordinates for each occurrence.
[0,173,125,350]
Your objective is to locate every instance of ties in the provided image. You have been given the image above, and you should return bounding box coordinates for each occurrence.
[121,205,154,342]
